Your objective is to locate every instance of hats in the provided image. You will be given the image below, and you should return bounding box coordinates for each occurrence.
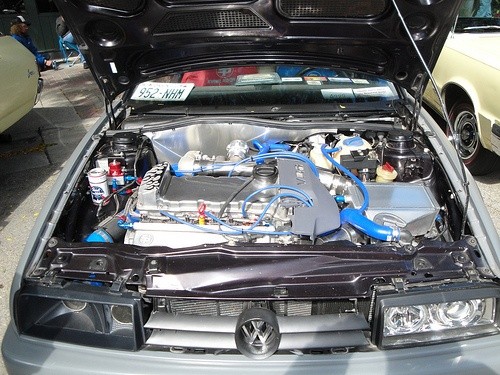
[9,15,32,26]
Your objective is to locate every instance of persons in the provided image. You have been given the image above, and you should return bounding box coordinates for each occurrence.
[8,16,53,68]
[55,15,89,69]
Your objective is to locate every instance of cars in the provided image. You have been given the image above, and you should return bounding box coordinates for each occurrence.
[423,1,500,178]
[0,0,500,375]
